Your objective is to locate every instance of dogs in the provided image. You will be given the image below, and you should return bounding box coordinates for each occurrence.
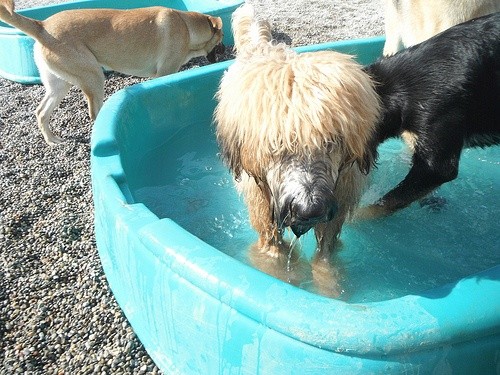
[0,0,223,145]
[216,0,386,294]
[347,0,499,224]
